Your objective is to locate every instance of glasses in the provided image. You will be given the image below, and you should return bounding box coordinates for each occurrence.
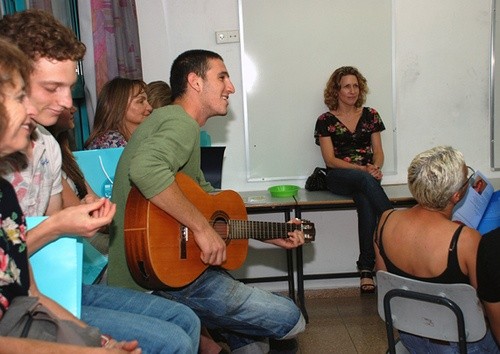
[459,165,476,191]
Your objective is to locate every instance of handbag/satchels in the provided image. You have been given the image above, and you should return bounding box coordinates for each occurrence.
[305,167,328,191]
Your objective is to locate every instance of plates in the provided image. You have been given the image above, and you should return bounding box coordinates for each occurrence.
[268,185,301,198]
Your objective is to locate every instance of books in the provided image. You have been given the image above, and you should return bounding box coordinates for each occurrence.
[451,170,500,235]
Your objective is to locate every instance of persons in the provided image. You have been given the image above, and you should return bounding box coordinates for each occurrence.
[44,103,101,210]
[314,66,392,294]
[475,228,500,348]
[144,80,171,113]
[0,35,142,354]
[82,77,152,150]
[0,10,201,354]
[106,49,307,354]
[372,146,500,354]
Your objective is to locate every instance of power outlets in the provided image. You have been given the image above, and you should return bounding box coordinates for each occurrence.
[216,30,239,43]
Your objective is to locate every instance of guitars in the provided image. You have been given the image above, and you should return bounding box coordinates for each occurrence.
[123,171,316,290]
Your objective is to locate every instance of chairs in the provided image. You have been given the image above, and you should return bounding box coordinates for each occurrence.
[376,270,488,354]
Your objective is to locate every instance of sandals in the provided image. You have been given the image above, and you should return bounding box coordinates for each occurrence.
[360,266,375,291]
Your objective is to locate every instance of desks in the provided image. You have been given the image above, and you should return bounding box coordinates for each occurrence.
[293,177,500,325]
[235,191,297,304]
